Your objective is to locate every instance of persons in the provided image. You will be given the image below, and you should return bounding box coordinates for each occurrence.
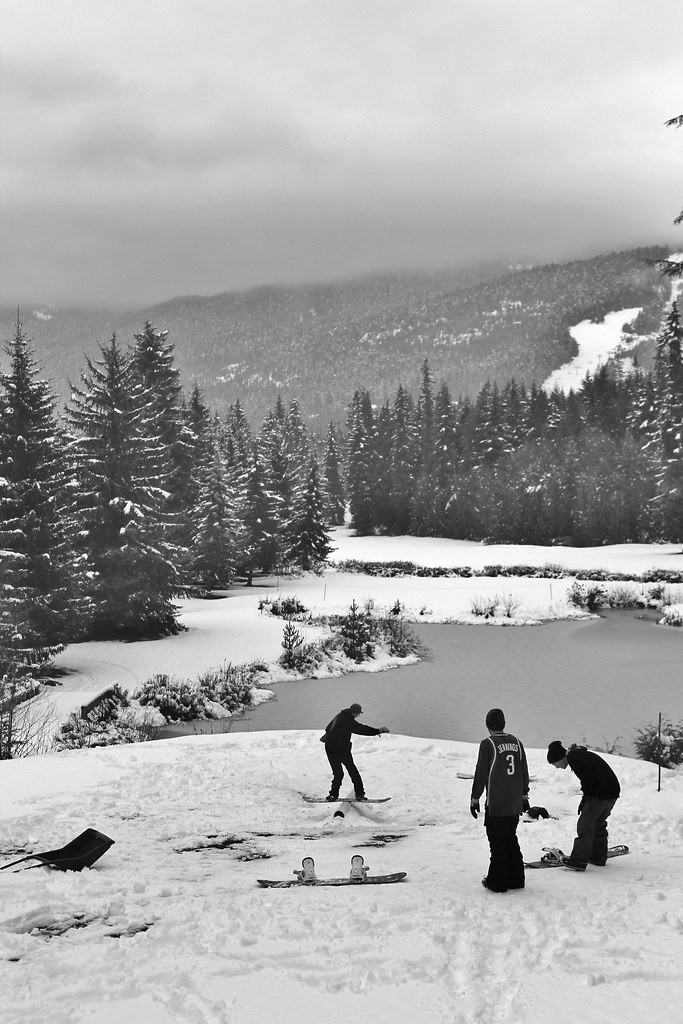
[320,702,390,802]
[547,739,621,871]
[469,708,531,893]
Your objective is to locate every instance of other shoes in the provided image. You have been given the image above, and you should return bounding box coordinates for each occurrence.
[482,878,487,887]
[326,795,336,800]
[356,796,368,802]
[560,856,587,871]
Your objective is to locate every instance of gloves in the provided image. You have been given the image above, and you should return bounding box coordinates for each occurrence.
[523,799,529,812]
[380,727,389,733]
[470,799,480,819]
[578,798,584,815]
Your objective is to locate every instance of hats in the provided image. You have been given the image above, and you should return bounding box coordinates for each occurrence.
[547,741,567,764]
[486,708,505,730]
[350,704,363,713]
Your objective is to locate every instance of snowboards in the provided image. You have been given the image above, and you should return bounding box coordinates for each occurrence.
[298,791,391,804]
[522,844,629,869]
[256,855,408,889]
